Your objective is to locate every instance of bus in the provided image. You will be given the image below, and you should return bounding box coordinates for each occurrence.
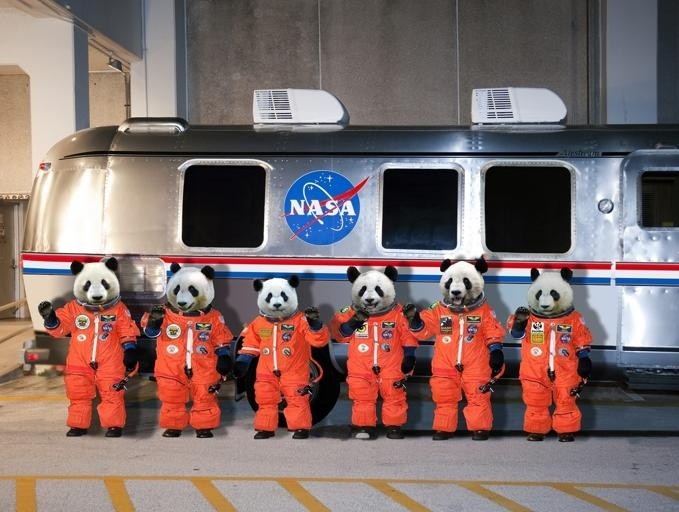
[21,87,678,428]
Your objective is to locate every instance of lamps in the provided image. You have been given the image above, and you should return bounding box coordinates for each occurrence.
[108,60,122,73]
